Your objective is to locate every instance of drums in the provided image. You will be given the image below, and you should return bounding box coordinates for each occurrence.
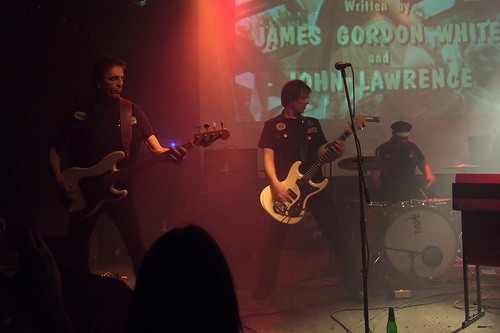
[347,200,392,249]
[384,201,459,279]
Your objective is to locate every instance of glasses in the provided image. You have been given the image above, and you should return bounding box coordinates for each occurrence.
[297,95,311,100]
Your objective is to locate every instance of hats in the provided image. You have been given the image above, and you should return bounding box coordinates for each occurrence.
[391,121,412,137]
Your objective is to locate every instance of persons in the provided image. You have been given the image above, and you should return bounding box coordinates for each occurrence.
[372,121,439,201]
[0,222,241,333]
[47,54,188,276]
[251,79,369,304]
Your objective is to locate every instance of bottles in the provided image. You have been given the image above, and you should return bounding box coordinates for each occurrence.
[387,307,397,333]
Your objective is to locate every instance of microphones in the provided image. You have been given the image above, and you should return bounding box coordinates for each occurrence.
[335,60,352,70]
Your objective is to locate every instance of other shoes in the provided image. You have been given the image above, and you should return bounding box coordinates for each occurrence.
[254,287,271,299]
[347,291,371,303]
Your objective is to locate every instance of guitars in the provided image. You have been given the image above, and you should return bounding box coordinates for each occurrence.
[259,115,368,224]
[60,120,231,220]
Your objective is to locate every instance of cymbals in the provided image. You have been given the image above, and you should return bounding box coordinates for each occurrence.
[338,155,396,172]
[448,164,481,168]
